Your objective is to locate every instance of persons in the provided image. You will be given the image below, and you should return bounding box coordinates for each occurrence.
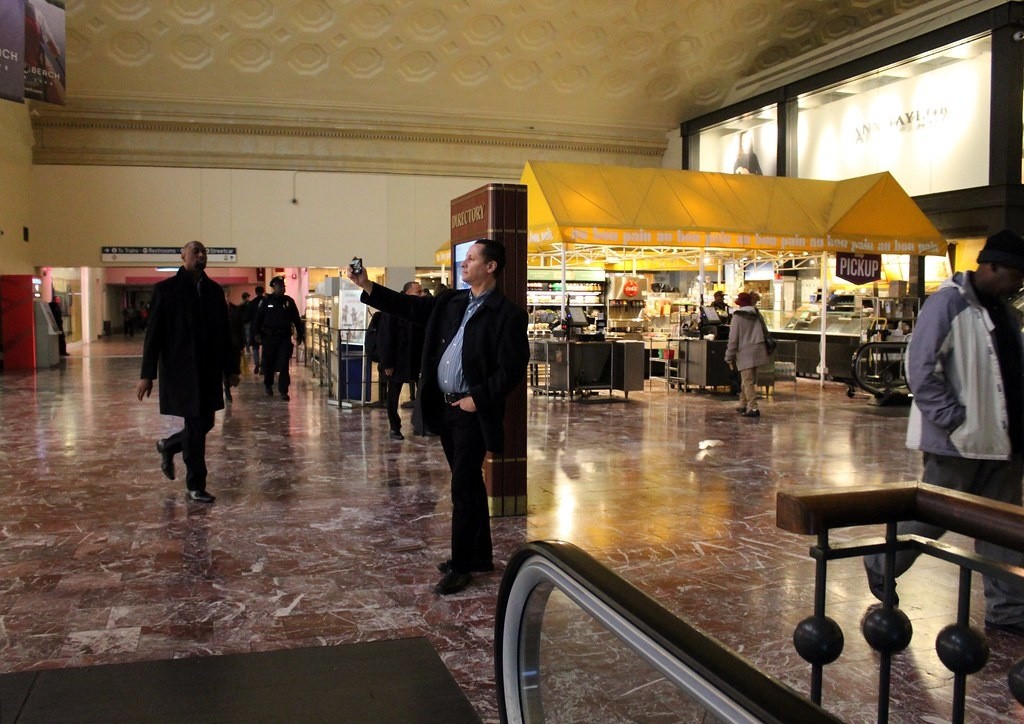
[251,276,303,400]
[238,292,251,355]
[136,240,238,502]
[50,296,69,356]
[248,287,265,374]
[711,291,728,308]
[223,287,245,400]
[724,292,768,416]
[121,301,149,335]
[348,239,531,594]
[864,229,1024,641]
[378,282,448,439]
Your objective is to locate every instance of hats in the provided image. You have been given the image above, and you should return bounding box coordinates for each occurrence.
[977,229,1024,269]
[735,293,751,306]
[715,291,727,296]
[270,276,284,287]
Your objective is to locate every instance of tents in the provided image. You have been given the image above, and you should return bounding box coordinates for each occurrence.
[522,161,947,398]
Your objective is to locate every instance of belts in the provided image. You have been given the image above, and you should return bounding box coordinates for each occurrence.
[443,391,469,403]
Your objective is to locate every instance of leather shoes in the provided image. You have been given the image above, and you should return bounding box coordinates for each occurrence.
[390,429,405,441]
[435,571,471,594]
[438,563,494,574]
[186,489,215,502]
[157,439,174,480]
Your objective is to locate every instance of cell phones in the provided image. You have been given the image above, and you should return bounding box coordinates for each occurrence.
[352,258,362,274]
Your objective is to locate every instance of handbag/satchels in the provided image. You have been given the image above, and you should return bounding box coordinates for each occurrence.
[765,332,777,355]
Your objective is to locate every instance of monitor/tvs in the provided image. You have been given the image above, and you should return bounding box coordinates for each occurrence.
[455,239,476,291]
[699,306,722,323]
[565,306,589,326]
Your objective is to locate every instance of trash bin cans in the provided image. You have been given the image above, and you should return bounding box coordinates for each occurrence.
[338,342,372,402]
[103,320,111,336]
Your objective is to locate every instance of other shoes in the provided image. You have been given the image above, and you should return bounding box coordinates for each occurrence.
[280,389,290,401]
[742,409,760,417]
[254,364,260,374]
[264,381,273,395]
[737,407,746,412]
[225,392,232,402]
[985,619,1024,637]
[862,558,899,605]
